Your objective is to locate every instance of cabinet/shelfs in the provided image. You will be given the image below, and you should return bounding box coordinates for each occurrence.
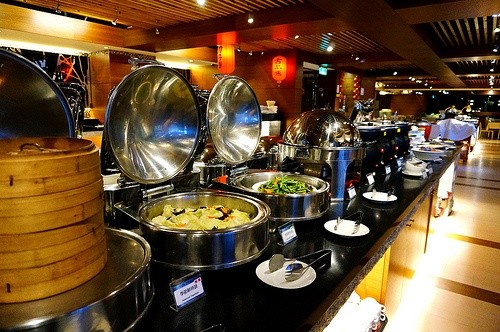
[136,136,465,332]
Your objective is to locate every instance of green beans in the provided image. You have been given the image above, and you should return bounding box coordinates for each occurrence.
[258,175,313,194]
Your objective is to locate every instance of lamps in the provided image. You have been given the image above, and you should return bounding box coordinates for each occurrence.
[237,48,240,51]
[491,60,495,63]
[352,53,359,61]
[494,26,500,32]
[489,66,494,72]
[408,76,432,89]
[318,67,327,75]
[248,51,252,55]
[112,18,118,25]
[493,44,499,52]
[156,28,159,34]
[55,4,62,13]
[248,13,253,24]
[392,67,397,75]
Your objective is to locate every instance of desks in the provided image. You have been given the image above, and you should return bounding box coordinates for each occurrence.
[488,122,500,129]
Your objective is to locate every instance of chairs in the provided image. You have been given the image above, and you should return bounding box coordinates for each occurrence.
[480,122,493,139]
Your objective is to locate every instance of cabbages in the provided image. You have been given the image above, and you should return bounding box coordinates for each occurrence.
[151,205,251,230]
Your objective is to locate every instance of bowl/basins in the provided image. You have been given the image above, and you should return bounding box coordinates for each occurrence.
[411,138,455,161]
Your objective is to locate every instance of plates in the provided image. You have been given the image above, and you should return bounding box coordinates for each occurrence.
[362,191,397,203]
[255,259,316,289]
[402,170,425,176]
[324,219,370,237]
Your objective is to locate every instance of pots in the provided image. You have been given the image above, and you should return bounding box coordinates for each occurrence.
[351,123,411,168]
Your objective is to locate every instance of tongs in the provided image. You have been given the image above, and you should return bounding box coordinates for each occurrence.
[371,185,397,198]
[333,209,363,234]
[267,248,331,280]
